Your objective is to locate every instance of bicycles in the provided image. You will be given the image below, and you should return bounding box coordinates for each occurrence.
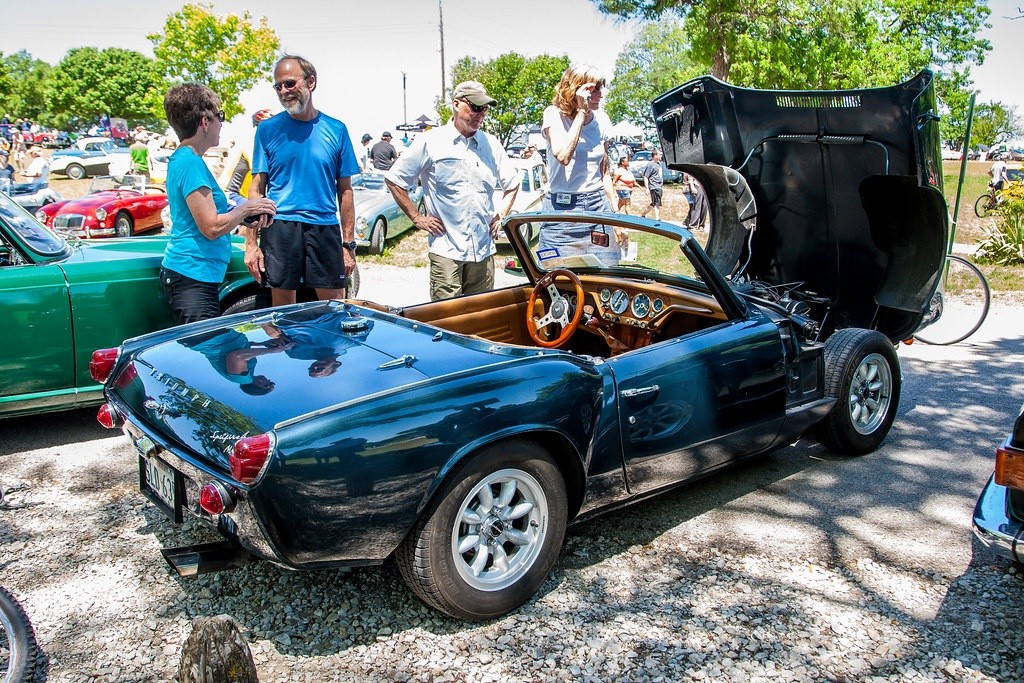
[911,212,990,345]
[974,180,1010,218]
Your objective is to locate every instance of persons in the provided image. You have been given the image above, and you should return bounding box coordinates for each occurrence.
[0,114,153,188]
[244,55,362,307]
[538,63,629,273]
[217,109,275,235]
[682,172,707,230]
[354,131,408,170]
[161,82,277,326]
[612,157,645,215]
[642,150,663,221]
[987,153,1011,203]
[523,143,545,162]
[385,81,521,302]
[176,308,374,396]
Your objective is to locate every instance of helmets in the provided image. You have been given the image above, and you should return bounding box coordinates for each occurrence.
[252,110,275,127]
[1001,152,1010,158]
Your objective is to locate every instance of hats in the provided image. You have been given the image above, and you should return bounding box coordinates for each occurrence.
[8,128,20,133]
[383,132,392,138]
[0,150,9,156]
[32,146,44,154]
[452,81,498,107]
[362,134,373,141]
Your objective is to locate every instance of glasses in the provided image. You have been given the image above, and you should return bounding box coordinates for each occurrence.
[273,79,306,91]
[308,367,332,372]
[459,99,490,112]
[214,110,225,121]
[595,80,606,89]
[265,382,275,388]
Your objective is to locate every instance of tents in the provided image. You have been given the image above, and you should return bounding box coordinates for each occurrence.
[396,114,439,132]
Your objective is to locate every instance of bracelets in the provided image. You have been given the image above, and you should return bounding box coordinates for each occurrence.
[411,214,420,221]
[577,108,587,115]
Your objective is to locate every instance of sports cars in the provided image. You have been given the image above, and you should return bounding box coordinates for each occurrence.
[90,67,951,627]
[0,188,318,421]
[335,171,428,257]
[34,174,170,239]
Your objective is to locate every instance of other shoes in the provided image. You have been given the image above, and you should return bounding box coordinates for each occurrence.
[688,228,699,232]
[20,171,25,176]
[701,228,705,232]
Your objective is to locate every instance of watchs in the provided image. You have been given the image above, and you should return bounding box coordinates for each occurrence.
[343,241,357,251]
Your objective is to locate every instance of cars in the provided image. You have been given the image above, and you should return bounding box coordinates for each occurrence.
[0,116,232,217]
[940,144,1013,161]
[492,144,550,247]
[607,140,684,185]
